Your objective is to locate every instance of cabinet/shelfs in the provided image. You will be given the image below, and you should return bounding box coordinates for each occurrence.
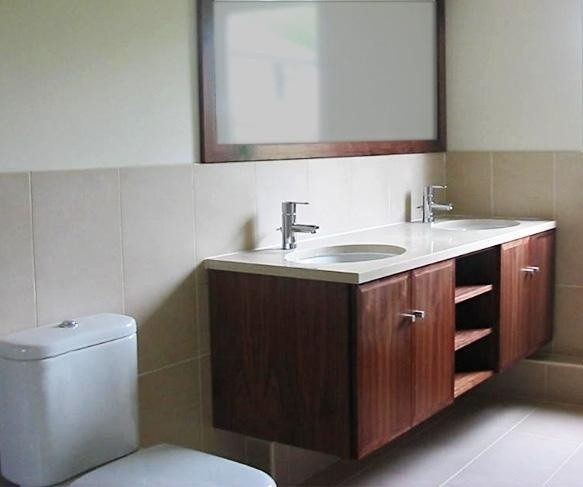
[455,245,501,399]
[500,227,558,375]
[206,257,455,461]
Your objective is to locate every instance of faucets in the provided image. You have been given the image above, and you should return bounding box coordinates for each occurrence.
[421,182,454,221]
[277,199,320,248]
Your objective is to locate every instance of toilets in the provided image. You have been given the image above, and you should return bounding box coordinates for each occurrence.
[0,311,279,486]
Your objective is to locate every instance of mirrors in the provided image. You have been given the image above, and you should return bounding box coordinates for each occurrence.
[196,0,445,163]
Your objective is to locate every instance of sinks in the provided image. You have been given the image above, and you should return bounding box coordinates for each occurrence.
[430,215,522,232]
[284,239,409,265]
